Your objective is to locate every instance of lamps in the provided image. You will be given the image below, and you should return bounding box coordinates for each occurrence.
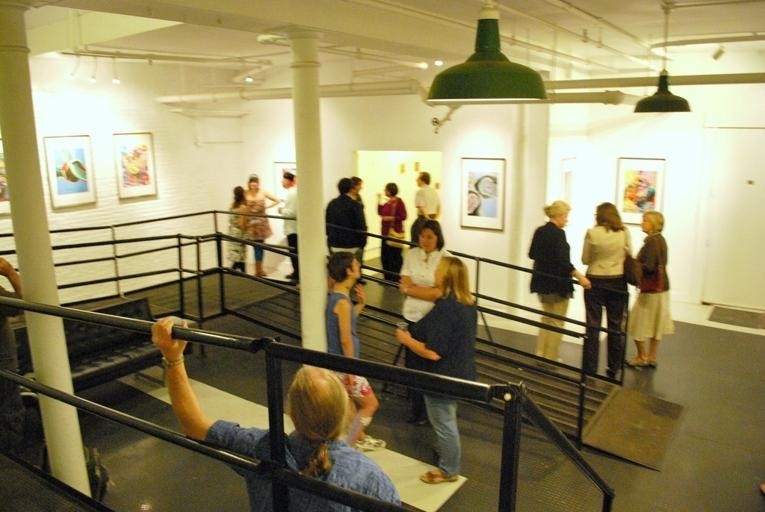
[426,1,547,102]
[634,14,690,112]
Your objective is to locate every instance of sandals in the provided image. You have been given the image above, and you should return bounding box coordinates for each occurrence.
[421,468,459,484]
[260,271,265,275]
[626,357,651,369]
[256,273,260,276]
[649,359,656,367]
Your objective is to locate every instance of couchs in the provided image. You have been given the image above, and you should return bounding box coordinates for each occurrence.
[13,298,193,394]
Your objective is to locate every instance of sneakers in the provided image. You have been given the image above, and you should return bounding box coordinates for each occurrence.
[354,437,387,452]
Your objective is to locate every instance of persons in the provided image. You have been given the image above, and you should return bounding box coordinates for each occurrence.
[376,183,407,282]
[0,259,23,430]
[529,201,591,370]
[326,251,387,453]
[417,172,439,220]
[227,186,263,278]
[391,255,475,484]
[230,175,279,279]
[326,176,367,304]
[278,172,297,287]
[344,177,364,210]
[626,211,674,368]
[584,202,633,382]
[150,315,402,512]
[397,221,457,422]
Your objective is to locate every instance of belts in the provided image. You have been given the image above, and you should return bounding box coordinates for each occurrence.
[420,214,437,219]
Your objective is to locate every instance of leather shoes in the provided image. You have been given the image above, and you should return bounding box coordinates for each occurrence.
[286,274,297,279]
[416,418,429,425]
[358,278,367,283]
[605,365,623,375]
[407,415,426,424]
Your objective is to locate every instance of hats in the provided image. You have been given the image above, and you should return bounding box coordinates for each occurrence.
[283,171,295,181]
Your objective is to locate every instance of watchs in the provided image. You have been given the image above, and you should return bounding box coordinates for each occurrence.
[161,357,184,368]
[403,288,405,297]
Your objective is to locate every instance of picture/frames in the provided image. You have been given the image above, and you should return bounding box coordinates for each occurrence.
[111,132,158,200]
[614,157,666,226]
[43,135,97,211]
[460,158,506,232]
[0,138,12,219]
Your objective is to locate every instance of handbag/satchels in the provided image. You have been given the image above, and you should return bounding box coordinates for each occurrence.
[638,235,665,294]
[386,198,405,249]
[382,341,415,417]
[622,227,641,289]
